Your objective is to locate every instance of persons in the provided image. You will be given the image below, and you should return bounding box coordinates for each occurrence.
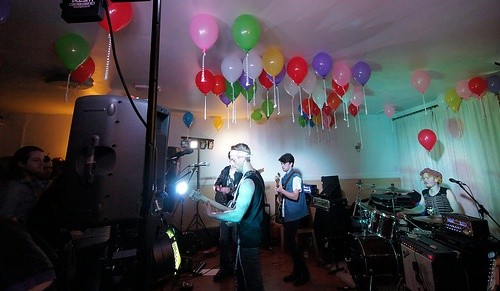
[276,153,310,286]
[202,142,266,291]
[401,168,463,217]
[0,145,83,291]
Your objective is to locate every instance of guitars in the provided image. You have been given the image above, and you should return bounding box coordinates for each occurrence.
[274,172,284,224]
[487,259,500,291]
[189,188,238,225]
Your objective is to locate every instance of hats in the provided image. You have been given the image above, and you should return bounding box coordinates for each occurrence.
[279,153,294,163]
[420,168,442,178]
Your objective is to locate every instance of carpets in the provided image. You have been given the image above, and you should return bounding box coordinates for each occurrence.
[328,258,356,287]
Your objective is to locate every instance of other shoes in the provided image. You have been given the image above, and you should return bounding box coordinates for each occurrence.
[284,272,310,286]
[212,268,235,282]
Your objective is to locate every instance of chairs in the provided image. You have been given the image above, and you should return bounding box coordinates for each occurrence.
[279,206,321,254]
[261,205,272,248]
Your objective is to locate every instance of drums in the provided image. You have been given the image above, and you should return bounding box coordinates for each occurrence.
[350,215,368,237]
[360,209,380,223]
[372,211,395,239]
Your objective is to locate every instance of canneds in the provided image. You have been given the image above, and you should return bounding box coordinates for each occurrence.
[304,251,308,258]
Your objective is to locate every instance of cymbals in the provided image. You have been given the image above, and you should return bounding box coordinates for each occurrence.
[351,180,365,185]
[378,184,409,194]
[367,184,380,190]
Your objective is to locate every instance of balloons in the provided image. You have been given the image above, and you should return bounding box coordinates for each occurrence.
[456,80,472,100]
[187,13,220,52]
[488,76,500,94]
[469,78,486,98]
[0,0,10,26]
[95,0,133,34]
[231,14,262,53]
[182,112,194,128]
[54,35,95,85]
[444,89,462,113]
[410,69,429,94]
[418,129,436,152]
[384,105,395,118]
[193,48,371,129]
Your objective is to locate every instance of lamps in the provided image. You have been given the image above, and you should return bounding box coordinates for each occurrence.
[181,138,214,149]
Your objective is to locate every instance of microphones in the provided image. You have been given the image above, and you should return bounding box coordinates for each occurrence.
[166,147,194,162]
[188,161,210,167]
[449,178,466,185]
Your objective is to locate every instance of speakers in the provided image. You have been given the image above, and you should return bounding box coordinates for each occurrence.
[313,204,357,265]
[400,236,496,291]
[55,93,171,224]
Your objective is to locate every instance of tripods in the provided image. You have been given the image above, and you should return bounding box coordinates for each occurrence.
[183,149,215,255]
